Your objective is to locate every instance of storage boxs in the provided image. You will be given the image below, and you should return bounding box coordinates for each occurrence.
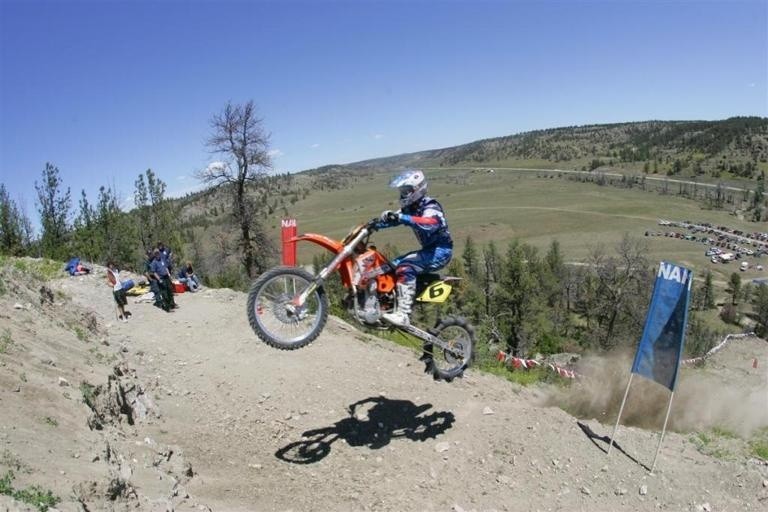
[172,280,187,292]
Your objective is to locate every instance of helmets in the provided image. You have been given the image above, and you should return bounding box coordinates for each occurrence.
[391,171,428,206]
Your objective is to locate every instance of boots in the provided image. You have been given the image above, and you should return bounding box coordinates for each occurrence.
[384,282,416,327]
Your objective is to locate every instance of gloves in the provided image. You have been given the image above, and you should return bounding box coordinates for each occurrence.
[381,210,399,225]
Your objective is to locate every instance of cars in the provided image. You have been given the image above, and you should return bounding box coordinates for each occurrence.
[646,219,768,271]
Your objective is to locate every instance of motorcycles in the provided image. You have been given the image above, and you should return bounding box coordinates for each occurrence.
[247,213,476,384]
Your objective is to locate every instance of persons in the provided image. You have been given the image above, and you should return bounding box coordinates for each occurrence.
[144,242,179,312]
[360,168,454,328]
[106,262,129,323]
[178,260,199,292]
